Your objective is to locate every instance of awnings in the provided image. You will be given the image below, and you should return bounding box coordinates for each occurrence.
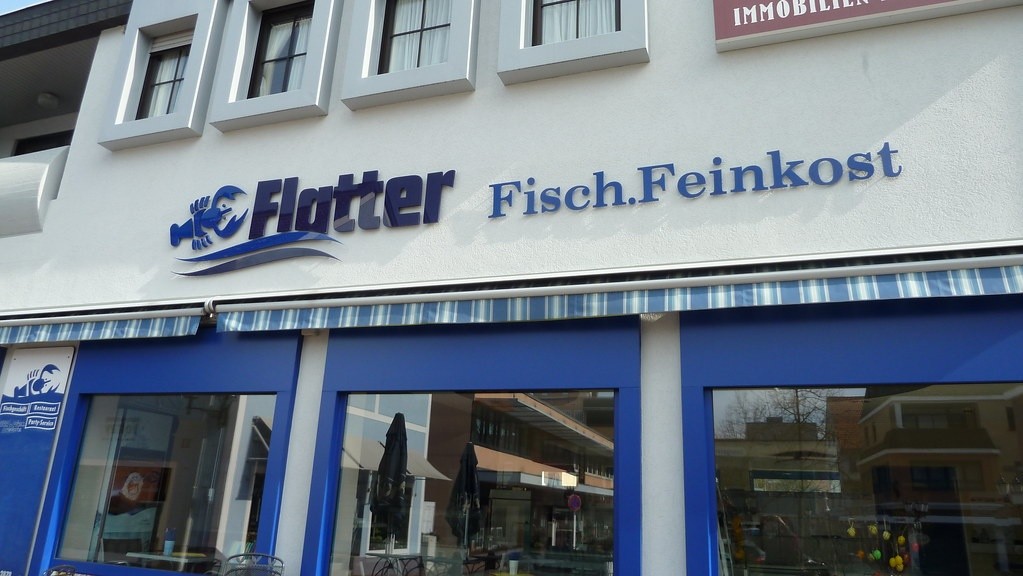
[245,415,452,481]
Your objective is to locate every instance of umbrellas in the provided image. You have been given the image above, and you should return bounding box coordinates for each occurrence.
[445,442,481,547]
[370,413,409,554]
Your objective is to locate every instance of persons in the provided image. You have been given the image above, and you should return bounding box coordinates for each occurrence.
[753,514,801,576]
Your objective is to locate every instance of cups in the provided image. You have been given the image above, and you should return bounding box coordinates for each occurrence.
[163,540,175,556]
[508,560,519,576]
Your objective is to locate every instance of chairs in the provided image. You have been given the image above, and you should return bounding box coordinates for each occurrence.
[207,553,283,576]
[103,538,143,567]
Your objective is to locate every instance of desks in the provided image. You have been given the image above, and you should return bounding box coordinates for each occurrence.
[729,564,832,576]
[125,550,216,573]
[363,549,503,576]
